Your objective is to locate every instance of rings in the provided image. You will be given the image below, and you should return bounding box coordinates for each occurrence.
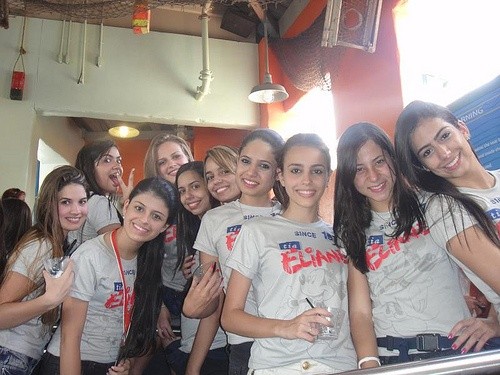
[309,326,312,334]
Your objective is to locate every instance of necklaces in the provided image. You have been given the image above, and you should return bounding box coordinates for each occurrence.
[239,198,274,222]
[372,208,395,230]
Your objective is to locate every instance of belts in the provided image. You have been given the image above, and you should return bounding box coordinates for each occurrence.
[377,334,457,352]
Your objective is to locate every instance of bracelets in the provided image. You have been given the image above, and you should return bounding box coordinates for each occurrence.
[358,357,381,369]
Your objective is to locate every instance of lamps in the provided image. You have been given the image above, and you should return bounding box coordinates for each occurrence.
[247,21,290,104]
[108,121,141,139]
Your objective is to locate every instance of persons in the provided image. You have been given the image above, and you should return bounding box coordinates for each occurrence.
[0,101,500,375]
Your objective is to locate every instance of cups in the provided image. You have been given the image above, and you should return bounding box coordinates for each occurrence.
[193,261,221,284]
[315,306,346,340]
[43,255,69,278]
